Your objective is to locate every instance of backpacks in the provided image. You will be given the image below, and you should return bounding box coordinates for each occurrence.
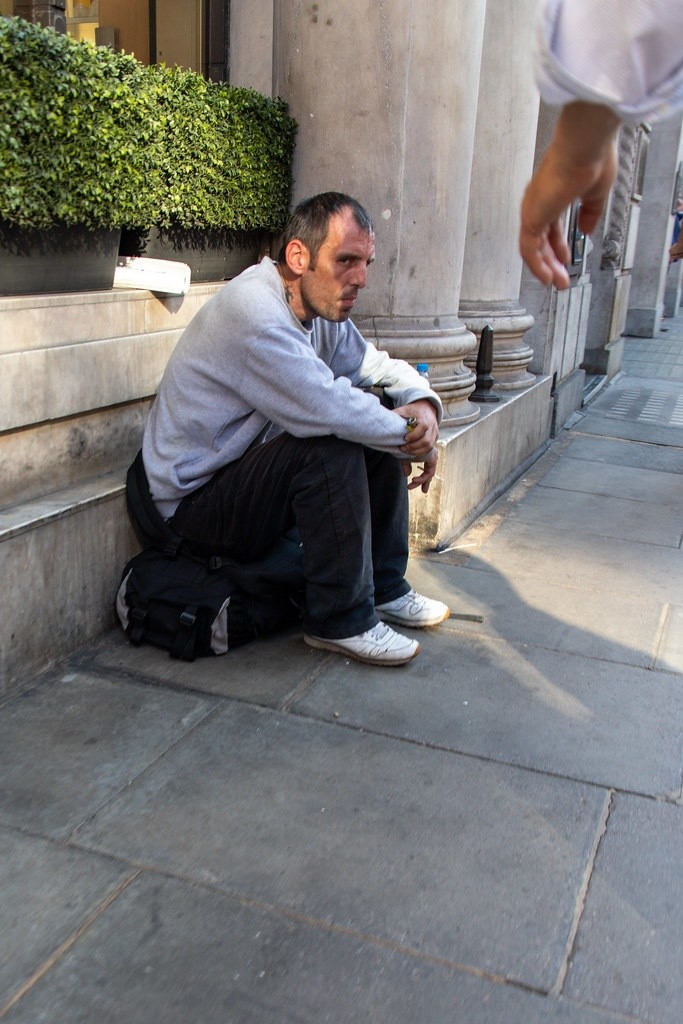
[115,523,307,663]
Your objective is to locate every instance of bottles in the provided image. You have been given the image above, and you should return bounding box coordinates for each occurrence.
[416,363,431,386]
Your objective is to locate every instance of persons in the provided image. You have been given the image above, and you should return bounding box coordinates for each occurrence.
[142,190,451,669]
[515,2,680,291]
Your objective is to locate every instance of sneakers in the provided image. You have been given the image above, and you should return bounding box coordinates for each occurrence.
[304,621,421,665]
[373,589,450,628]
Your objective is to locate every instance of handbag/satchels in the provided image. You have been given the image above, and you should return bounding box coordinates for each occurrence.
[124,448,176,551]
